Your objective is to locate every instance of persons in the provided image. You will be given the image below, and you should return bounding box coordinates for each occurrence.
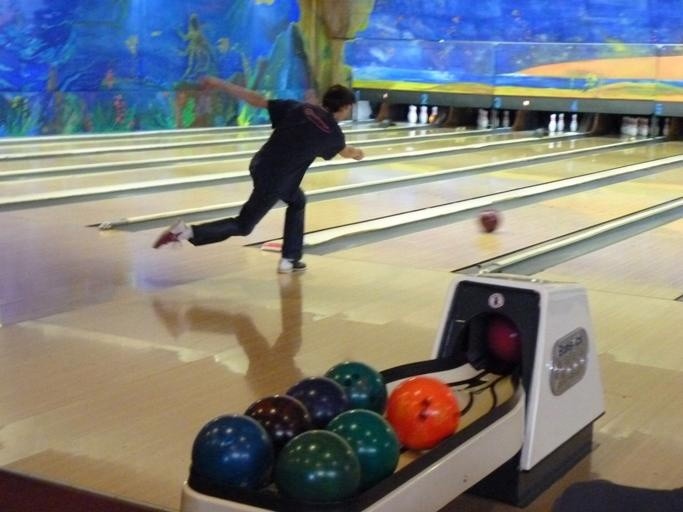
[152,76,364,274]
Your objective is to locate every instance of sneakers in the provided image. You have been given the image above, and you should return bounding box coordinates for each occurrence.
[152,218,186,249]
[278,259,309,274]
